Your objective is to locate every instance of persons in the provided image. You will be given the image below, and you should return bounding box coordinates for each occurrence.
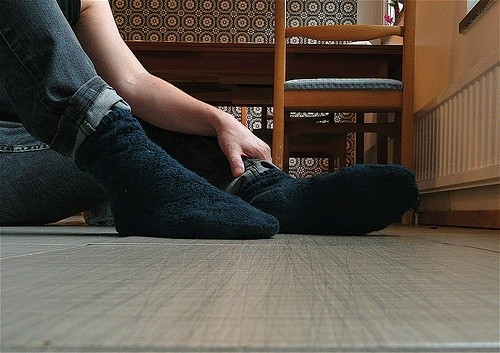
[0,0,420,240]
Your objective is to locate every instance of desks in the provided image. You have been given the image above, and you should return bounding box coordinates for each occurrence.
[125,41,405,177]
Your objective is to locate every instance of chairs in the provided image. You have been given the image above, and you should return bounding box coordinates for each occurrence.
[273,0,416,225]
[283,109,335,173]
